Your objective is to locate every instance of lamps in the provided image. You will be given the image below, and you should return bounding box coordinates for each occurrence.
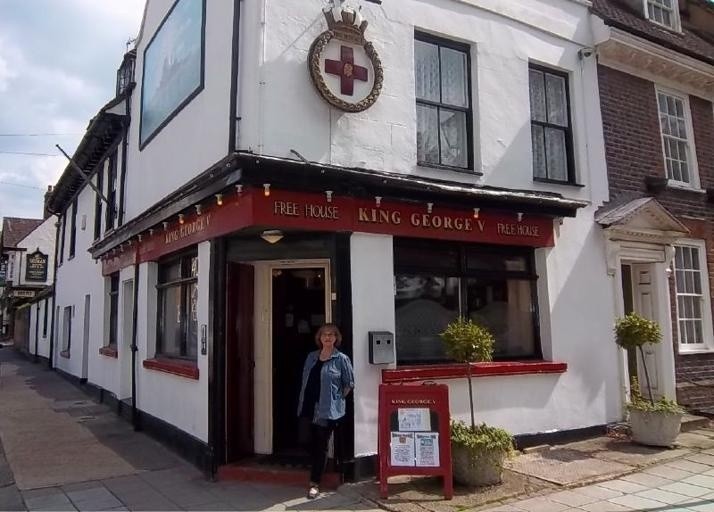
[177,184,244,225]
[260,230,285,244]
[263,183,526,224]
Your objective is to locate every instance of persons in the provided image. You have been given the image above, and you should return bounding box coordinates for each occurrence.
[294,324,356,502]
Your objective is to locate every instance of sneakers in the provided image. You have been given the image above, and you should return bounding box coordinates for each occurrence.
[308,486,320,499]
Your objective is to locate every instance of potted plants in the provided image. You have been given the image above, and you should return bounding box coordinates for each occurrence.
[437,314,514,488]
[610,311,686,448]
